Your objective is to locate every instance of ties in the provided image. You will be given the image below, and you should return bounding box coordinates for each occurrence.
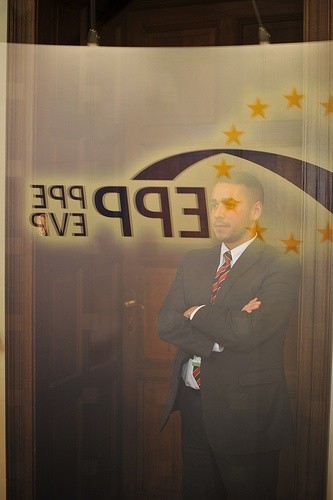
[193,251,232,388]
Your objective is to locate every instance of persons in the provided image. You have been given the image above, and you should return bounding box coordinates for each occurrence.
[153,168,306,500]
